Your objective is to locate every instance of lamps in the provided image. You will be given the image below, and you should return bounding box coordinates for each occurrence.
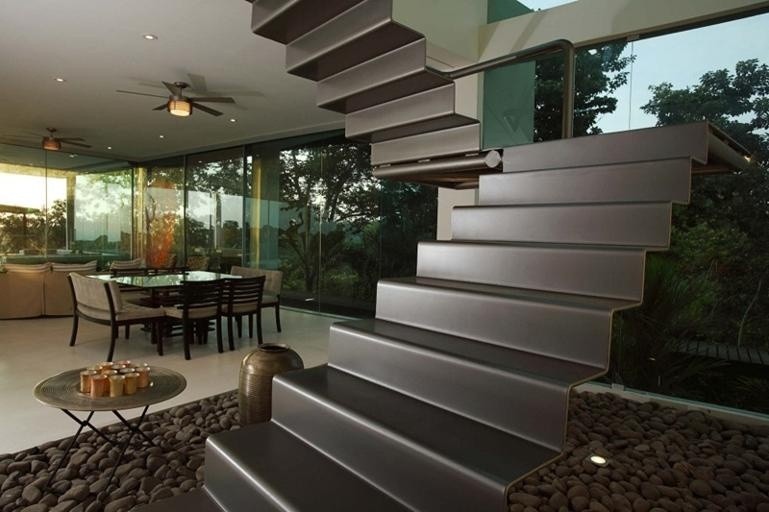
[168,82,191,117]
[42,128,60,150]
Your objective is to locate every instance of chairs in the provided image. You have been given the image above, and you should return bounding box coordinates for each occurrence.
[67,266,283,362]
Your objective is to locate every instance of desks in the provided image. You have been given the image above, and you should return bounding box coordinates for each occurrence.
[34,365,187,491]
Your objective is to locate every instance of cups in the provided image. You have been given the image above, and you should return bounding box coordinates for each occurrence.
[79,360,151,400]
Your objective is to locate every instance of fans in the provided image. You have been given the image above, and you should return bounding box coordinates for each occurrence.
[3,128,92,149]
[115,81,235,118]
